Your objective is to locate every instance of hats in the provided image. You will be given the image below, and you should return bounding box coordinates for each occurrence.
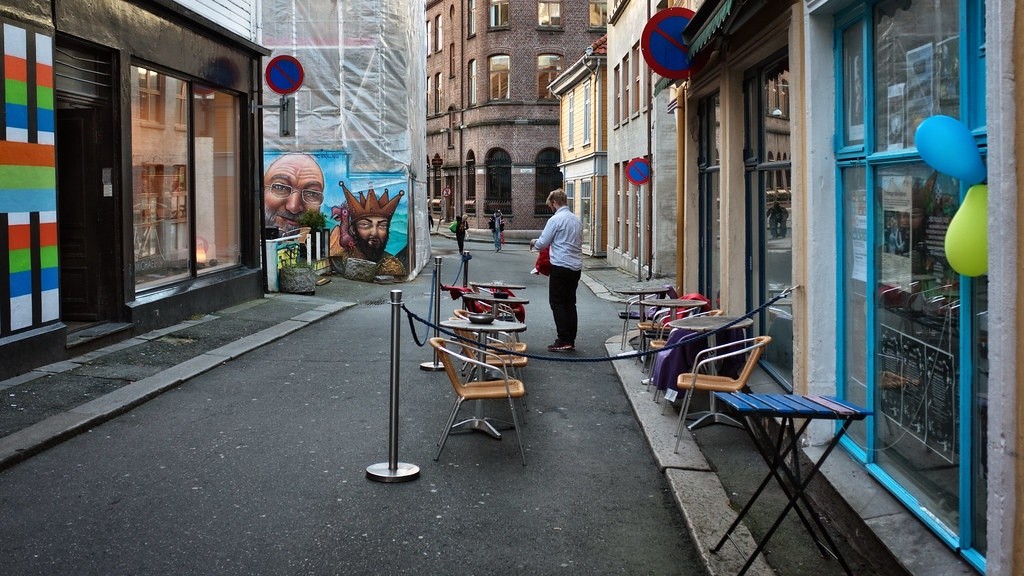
[495,210,501,213]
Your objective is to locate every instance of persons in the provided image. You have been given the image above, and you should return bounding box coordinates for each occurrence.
[767,202,789,239]
[456,214,469,255]
[429,215,434,231]
[529,189,583,352]
[488,209,505,253]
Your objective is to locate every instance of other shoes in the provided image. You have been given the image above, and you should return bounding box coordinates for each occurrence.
[548,341,575,352]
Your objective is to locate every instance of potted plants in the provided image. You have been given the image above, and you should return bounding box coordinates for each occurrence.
[298,209,326,258]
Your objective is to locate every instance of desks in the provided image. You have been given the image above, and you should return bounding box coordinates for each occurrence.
[469,282,527,293]
[943,286,986,299]
[439,319,527,440]
[638,299,707,321]
[708,390,875,576]
[669,319,754,430]
[913,274,945,297]
[613,288,668,362]
[462,293,529,317]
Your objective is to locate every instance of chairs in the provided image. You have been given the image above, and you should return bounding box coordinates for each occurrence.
[672,335,772,454]
[851,337,911,435]
[877,280,988,348]
[429,284,527,467]
[617,284,723,416]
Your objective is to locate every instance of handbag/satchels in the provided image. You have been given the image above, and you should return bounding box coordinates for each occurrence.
[449,222,458,233]
[500,231,504,245]
[464,220,470,242]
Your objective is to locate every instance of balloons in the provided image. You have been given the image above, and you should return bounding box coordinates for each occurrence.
[945,185,988,277]
[914,114,987,184]
